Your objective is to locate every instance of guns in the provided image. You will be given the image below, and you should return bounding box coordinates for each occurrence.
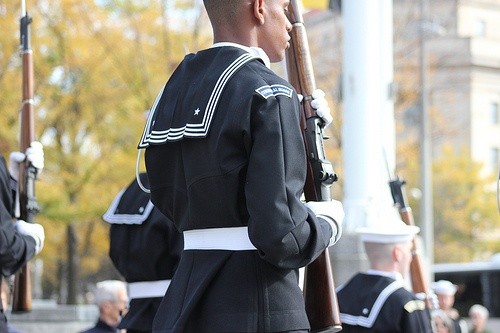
[385,160,435,313]
[285,0,344,333]
[10,0,41,315]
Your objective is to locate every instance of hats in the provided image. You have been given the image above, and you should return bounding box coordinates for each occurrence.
[94,277,128,302]
[432,280,463,295]
[469,304,489,318]
[353,205,420,240]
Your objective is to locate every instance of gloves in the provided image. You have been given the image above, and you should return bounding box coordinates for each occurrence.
[15,221,50,250]
[9,138,48,183]
[417,292,440,313]
[309,195,346,240]
[289,85,338,129]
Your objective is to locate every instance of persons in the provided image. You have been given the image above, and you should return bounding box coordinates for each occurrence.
[429,281,492,333]
[77,280,129,333]
[101,171,183,332]
[136,1,344,332]
[335,223,432,333]
[0,143,44,333]
